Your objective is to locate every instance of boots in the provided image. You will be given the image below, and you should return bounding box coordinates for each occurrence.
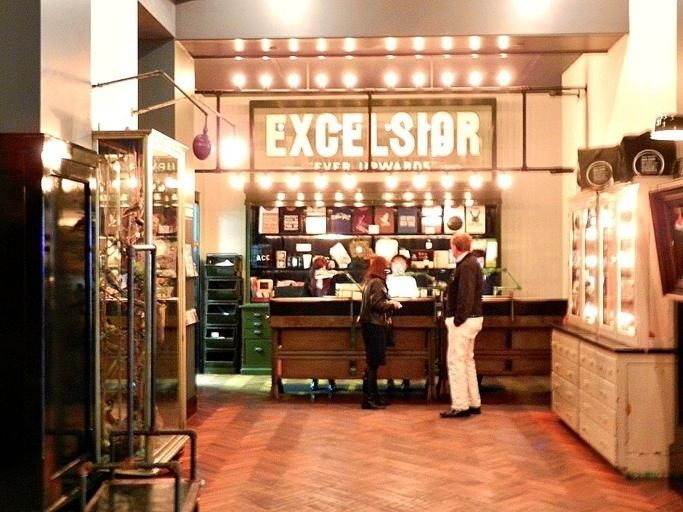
[362,381,389,409]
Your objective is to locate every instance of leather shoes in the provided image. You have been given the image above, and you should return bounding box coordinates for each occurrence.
[471,406,482,415]
[440,406,470,417]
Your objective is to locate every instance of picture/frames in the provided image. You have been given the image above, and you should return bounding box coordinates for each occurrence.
[647,178,682,302]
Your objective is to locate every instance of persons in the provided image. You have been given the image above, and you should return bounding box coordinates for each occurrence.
[438,232,483,416]
[360,260,404,409]
[384,254,419,297]
[303,260,337,390]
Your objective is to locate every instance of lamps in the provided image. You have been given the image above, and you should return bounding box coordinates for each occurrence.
[650,117,683,141]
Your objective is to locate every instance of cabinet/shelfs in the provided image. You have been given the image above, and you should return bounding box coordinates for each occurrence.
[238,198,502,374]
[548,176,675,482]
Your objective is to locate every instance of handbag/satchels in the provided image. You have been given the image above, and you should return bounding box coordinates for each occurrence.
[386,322,394,346]
